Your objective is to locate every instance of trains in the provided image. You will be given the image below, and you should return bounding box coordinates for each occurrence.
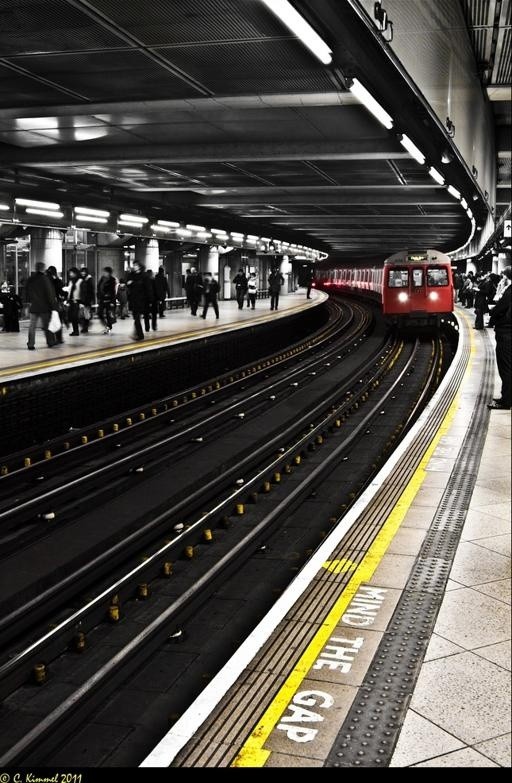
[308,243,456,334]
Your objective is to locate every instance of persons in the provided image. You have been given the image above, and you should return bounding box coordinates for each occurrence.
[25,264,171,350]
[452,269,498,331]
[306,271,315,297]
[485,268,512,409]
[233,269,259,309]
[186,269,220,321]
[269,269,284,310]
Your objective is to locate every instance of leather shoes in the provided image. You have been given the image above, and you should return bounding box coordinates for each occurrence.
[486,397,511,410]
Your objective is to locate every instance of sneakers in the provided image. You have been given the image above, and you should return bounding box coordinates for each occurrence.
[68,323,111,336]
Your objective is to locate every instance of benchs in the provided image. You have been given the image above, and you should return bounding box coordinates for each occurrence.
[164,296,187,309]
[257,291,269,299]
